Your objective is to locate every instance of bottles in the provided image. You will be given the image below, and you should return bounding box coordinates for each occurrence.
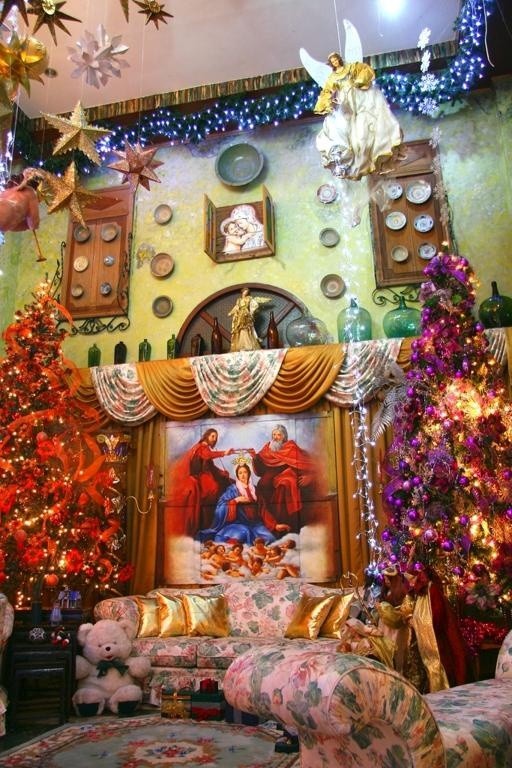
[188,331,205,356]
[112,340,127,365]
[135,337,152,362]
[88,342,102,368]
[164,334,179,360]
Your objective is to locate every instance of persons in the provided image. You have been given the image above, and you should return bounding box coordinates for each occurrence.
[315,50,403,179]
[164,423,320,583]
[219,204,265,254]
[228,288,263,352]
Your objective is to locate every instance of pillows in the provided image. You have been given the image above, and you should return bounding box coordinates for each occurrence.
[283,590,357,642]
[135,591,230,639]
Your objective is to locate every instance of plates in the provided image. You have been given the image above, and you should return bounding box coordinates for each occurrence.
[315,183,336,203]
[69,224,119,297]
[152,204,171,227]
[320,274,346,299]
[215,141,267,188]
[319,228,340,248]
[149,252,174,278]
[386,179,437,267]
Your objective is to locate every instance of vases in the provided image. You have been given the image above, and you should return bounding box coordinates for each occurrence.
[167,333,179,361]
[113,341,127,364]
[88,343,100,366]
[190,333,204,357]
[139,339,151,362]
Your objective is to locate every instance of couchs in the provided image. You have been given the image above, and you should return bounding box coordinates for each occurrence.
[91,587,406,694]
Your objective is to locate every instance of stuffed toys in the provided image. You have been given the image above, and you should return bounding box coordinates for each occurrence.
[71,618,152,718]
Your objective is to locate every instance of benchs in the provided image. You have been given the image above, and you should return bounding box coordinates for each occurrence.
[224,628,512,767]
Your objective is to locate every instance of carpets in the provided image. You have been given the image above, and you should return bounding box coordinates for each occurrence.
[0,716,291,768]
